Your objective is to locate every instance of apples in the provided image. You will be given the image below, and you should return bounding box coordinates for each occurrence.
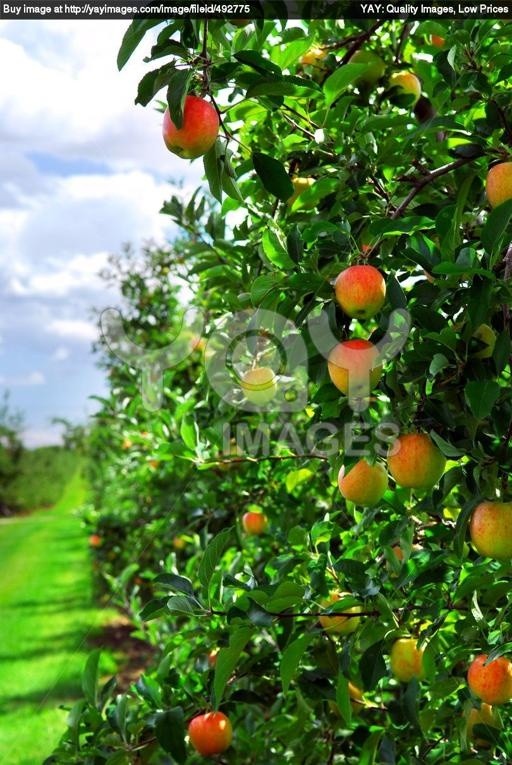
[87,35,512,759]
